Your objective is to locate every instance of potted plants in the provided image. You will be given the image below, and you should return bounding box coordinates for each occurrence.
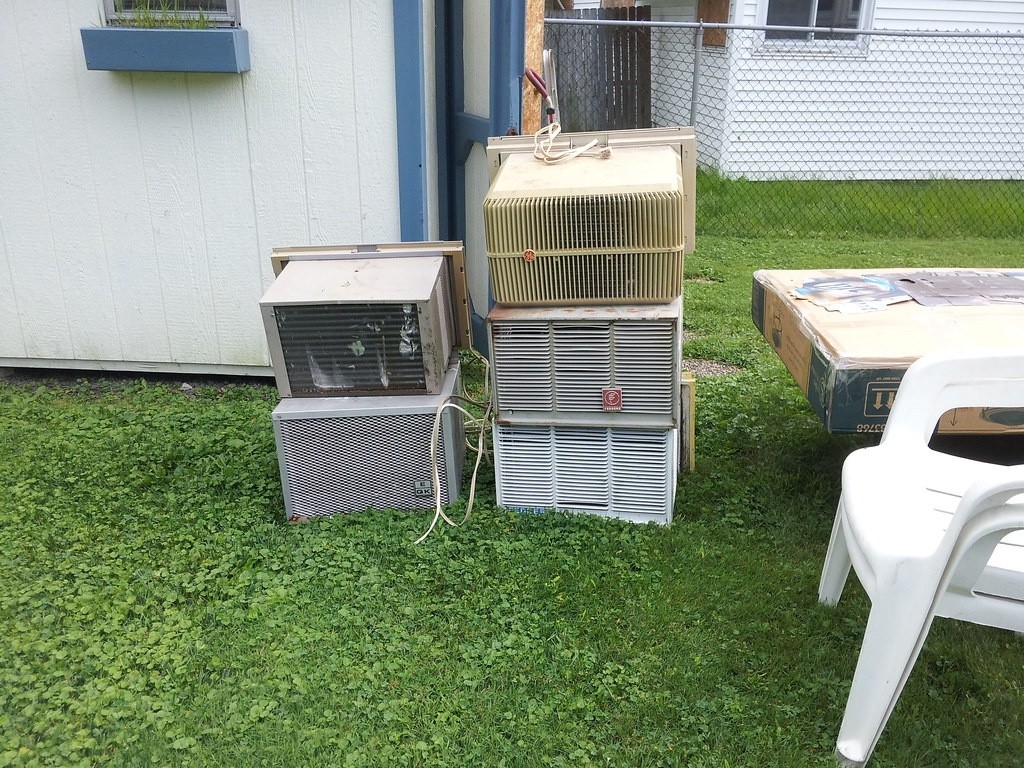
[79,1,251,73]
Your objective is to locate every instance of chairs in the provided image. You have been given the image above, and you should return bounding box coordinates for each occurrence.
[817,349,1024,768]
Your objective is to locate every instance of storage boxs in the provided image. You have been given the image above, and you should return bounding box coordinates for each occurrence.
[752,267,1024,435]
